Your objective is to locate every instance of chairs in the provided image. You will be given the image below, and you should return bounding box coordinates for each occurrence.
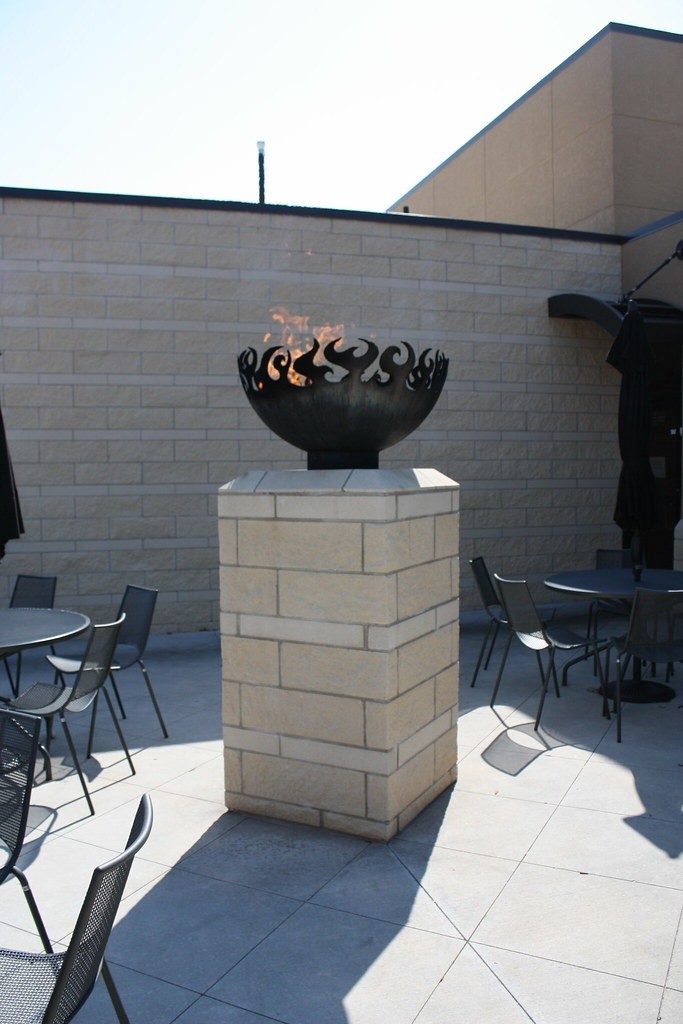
[489,572,611,732]
[0,574,170,956]
[0,792,153,1024]
[601,587,683,744]
[584,548,648,660]
[469,557,554,695]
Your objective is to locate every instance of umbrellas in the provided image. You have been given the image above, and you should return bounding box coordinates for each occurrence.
[606,299,664,570]
[0,408,27,558]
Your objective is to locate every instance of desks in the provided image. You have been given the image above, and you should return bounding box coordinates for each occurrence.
[544,566,683,704]
[0,606,90,766]
